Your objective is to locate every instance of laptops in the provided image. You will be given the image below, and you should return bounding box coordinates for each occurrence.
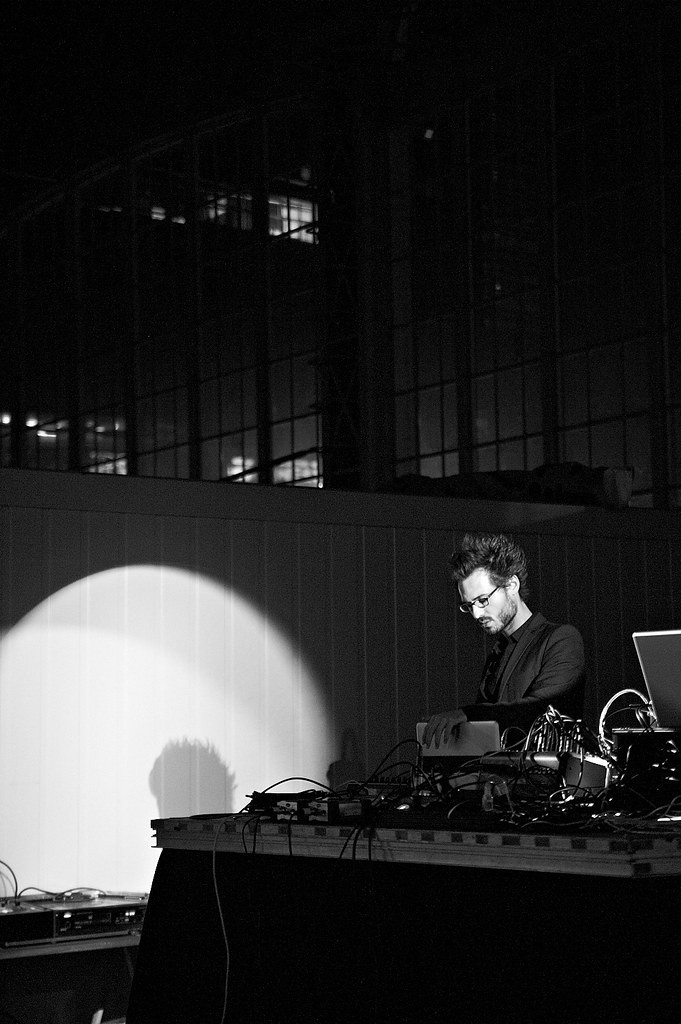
[416,721,501,772]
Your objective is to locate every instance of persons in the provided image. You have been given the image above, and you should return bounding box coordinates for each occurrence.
[422,531,586,752]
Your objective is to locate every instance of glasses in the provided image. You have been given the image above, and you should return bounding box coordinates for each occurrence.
[459,585,500,613]
